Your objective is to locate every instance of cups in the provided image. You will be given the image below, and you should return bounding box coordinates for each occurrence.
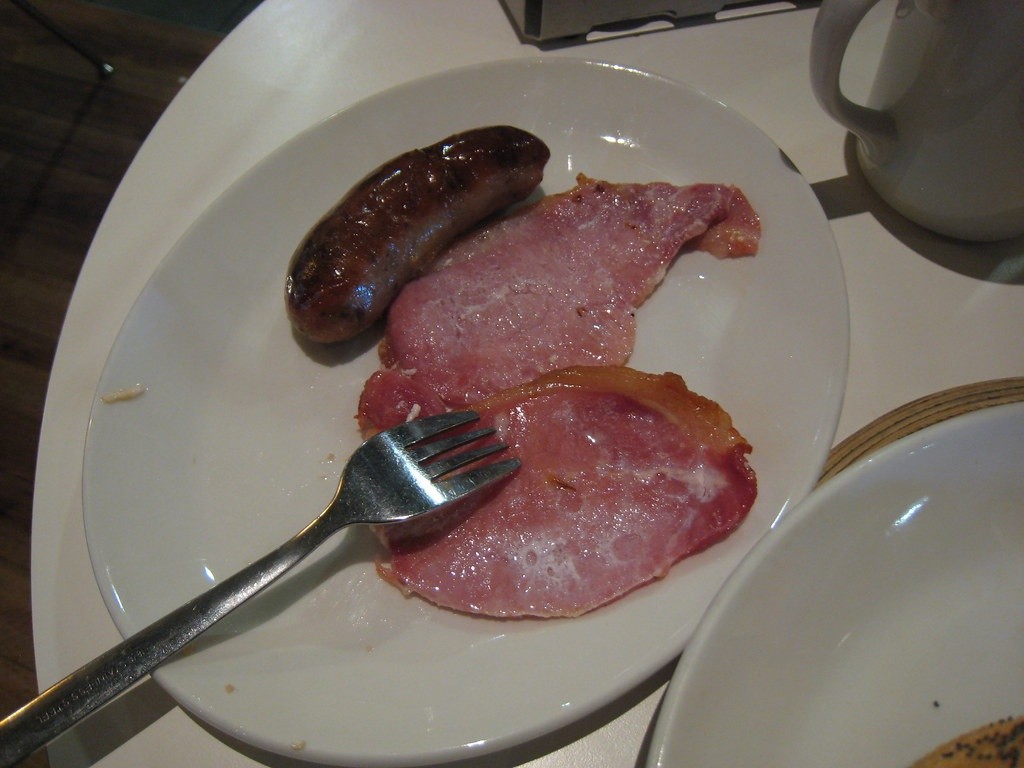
[808,0,1024,243]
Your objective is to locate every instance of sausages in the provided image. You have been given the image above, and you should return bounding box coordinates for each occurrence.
[283,125,552,345]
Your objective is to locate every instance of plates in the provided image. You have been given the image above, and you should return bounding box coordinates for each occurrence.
[643,398,1024,768]
[80,57,851,768]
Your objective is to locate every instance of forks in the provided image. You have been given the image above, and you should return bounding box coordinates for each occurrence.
[0,408,522,767]
